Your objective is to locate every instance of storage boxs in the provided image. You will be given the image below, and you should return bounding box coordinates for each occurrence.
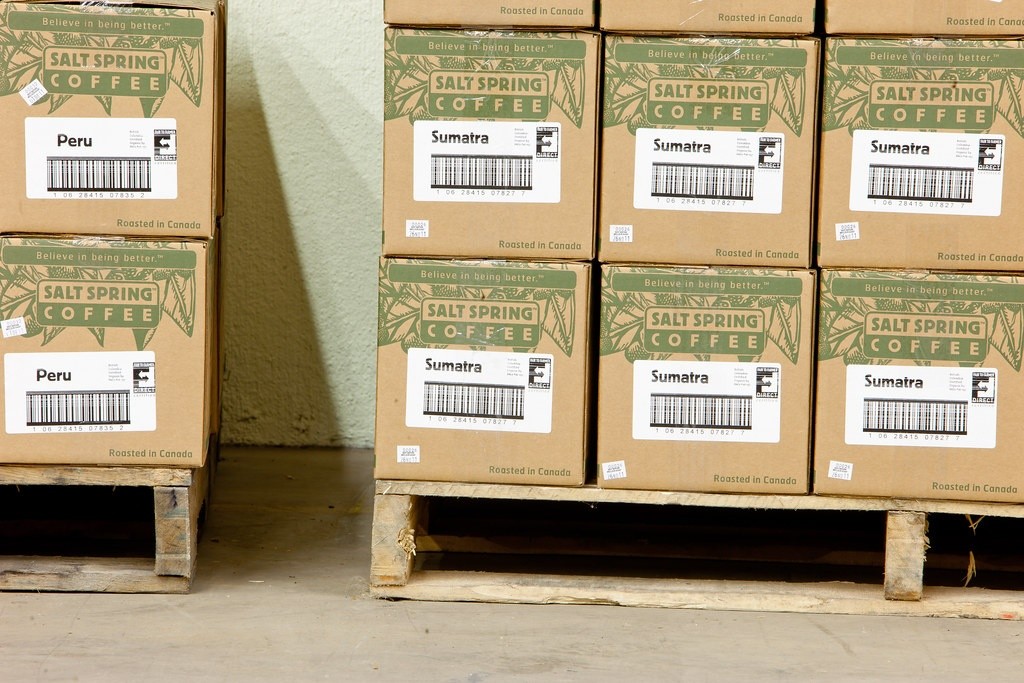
[373,2,1024,505]
[0,0,227,472]
